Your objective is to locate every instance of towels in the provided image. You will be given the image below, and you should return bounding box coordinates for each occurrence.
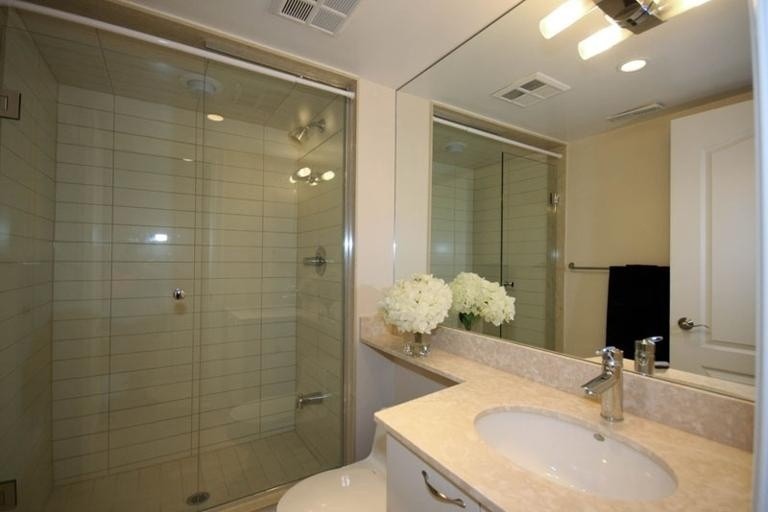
[606,264,670,361]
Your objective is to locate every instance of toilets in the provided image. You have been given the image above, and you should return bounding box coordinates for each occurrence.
[275,412,400,512]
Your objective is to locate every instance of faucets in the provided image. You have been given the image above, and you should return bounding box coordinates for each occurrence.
[581,347,625,422]
[635,335,670,377]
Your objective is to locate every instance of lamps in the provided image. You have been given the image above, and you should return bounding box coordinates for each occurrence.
[538,0,716,61]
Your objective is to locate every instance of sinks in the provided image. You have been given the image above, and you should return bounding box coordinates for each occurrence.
[474,404,680,504]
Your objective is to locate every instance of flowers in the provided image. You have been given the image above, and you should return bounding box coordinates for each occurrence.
[376,272,455,343]
[448,270,517,333]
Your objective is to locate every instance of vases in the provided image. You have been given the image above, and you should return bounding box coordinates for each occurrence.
[405,342,431,358]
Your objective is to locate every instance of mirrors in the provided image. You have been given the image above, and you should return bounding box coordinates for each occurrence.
[394,0,758,406]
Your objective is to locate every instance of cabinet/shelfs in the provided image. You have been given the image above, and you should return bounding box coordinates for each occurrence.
[385,436,491,509]
